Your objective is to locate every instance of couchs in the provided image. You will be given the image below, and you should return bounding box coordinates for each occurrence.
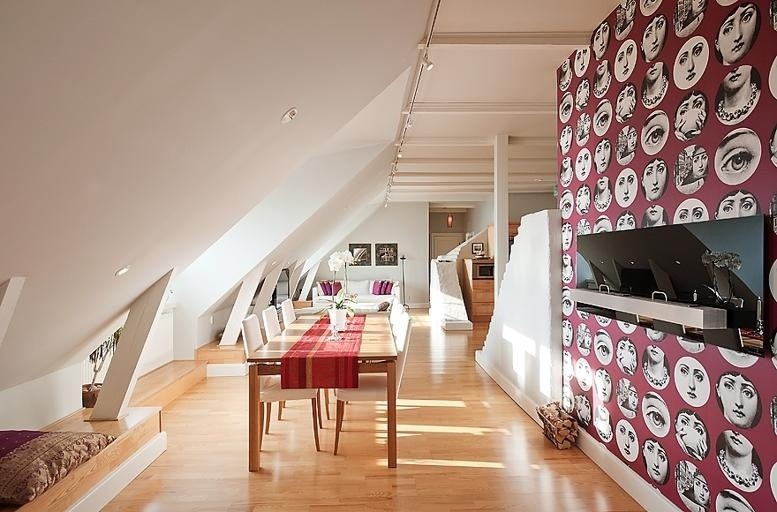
[311,279,401,308]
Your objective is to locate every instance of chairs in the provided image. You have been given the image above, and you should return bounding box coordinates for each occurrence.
[240,299,412,471]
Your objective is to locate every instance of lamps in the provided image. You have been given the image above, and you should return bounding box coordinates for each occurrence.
[447,213,453,228]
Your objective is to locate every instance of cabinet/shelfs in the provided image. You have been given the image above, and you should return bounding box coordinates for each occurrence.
[463,258,494,322]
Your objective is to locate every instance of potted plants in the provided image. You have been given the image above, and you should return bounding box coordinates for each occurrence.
[82,327,123,408]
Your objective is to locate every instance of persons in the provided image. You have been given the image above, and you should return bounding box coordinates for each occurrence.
[557,2,777,510]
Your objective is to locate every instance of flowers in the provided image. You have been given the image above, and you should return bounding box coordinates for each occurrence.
[328,251,344,310]
[342,249,354,308]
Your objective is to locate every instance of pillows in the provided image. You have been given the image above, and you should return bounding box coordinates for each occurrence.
[0,431,116,503]
[318,281,342,296]
[372,280,393,295]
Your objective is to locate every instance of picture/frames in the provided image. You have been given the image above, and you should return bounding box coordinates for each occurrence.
[349,243,372,266]
[375,243,398,266]
[472,243,484,254]
